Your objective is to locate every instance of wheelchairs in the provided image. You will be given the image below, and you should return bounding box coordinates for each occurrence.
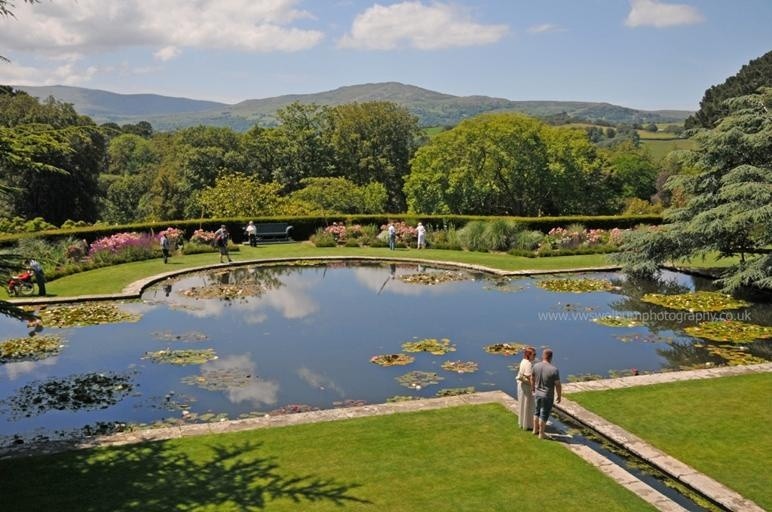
[5,268,34,298]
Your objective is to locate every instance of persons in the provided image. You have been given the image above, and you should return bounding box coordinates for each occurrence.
[388,224,396,250]
[415,222,427,249]
[161,233,171,263]
[214,225,232,263]
[25,260,46,296]
[246,221,257,247]
[531,348,561,440]
[516,347,536,430]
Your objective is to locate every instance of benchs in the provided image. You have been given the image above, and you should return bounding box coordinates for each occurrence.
[254,222,295,242]
